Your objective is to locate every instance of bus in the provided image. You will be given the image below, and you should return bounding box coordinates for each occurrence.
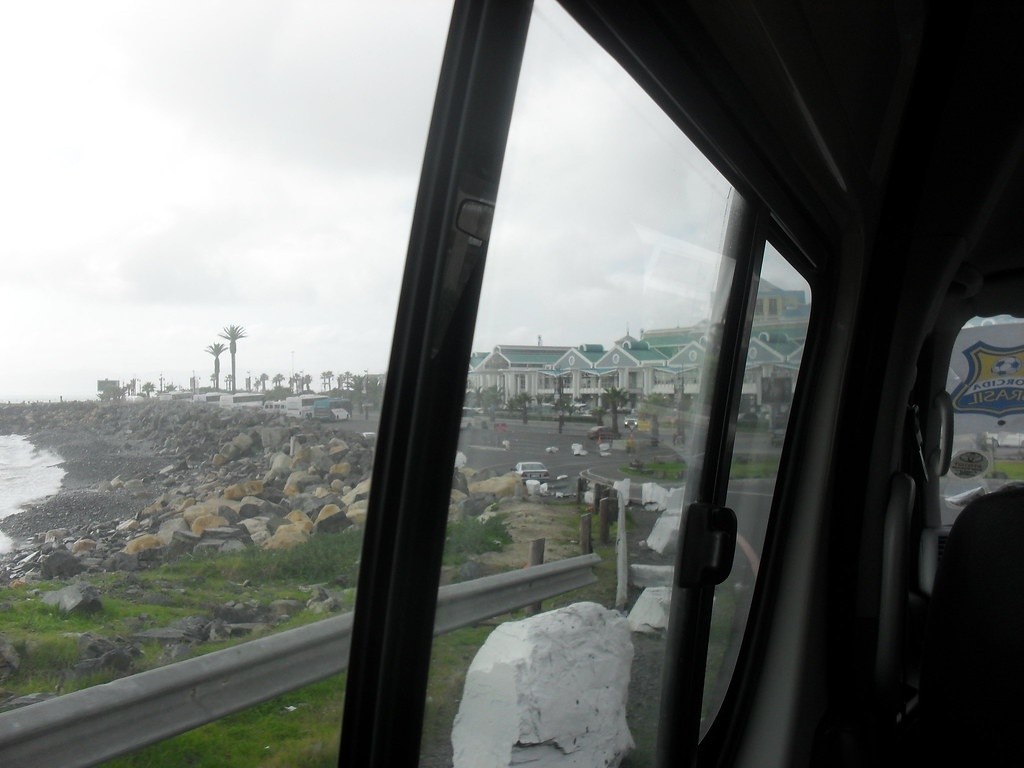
[125,392,352,422]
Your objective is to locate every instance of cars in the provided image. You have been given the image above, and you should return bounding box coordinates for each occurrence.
[624,415,638,429]
[737,413,758,426]
[510,462,549,482]
[587,426,621,440]
